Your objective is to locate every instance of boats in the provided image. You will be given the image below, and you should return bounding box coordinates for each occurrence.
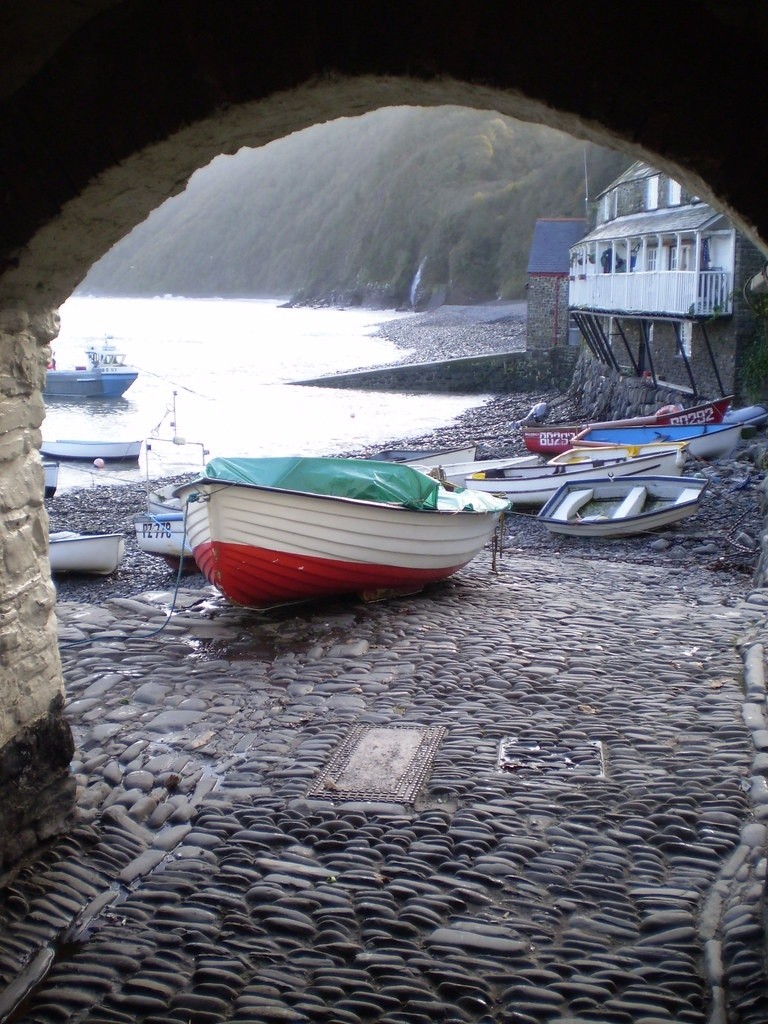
[413,455,538,489]
[534,475,711,536]
[463,439,693,506]
[41,463,61,499]
[39,332,139,399]
[36,437,143,461]
[132,445,516,607]
[569,421,744,459]
[523,393,737,454]
[45,530,129,579]
